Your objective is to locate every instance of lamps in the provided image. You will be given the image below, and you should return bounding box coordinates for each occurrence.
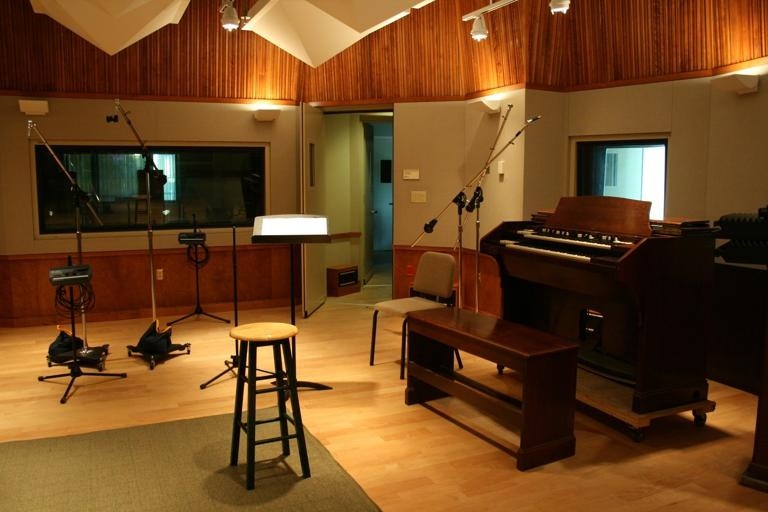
[468,100,501,115]
[255,109,281,122]
[546,0,571,15]
[470,13,490,43]
[711,74,761,96]
[216,0,242,35]
[19,99,50,116]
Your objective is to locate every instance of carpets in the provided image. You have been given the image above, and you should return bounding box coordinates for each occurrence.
[0,405,380,512]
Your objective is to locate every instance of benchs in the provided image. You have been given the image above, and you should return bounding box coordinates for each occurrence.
[403,304,581,473]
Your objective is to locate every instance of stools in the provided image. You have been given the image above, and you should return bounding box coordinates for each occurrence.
[227,320,312,491]
[128,194,153,227]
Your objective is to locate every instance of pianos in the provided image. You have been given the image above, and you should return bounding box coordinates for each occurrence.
[479,194,715,416]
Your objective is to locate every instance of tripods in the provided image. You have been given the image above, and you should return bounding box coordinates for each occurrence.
[37,287,127,406]
[41,130,111,371]
[167,245,231,333]
[125,116,192,371]
[255,244,333,397]
[201,228,286,390]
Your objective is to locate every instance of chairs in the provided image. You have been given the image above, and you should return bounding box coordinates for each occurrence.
[370,251,466,380]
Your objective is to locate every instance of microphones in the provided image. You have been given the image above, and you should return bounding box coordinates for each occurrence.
[27,120,32,137]
[113,98,120,122]
[466,186,484,213]
